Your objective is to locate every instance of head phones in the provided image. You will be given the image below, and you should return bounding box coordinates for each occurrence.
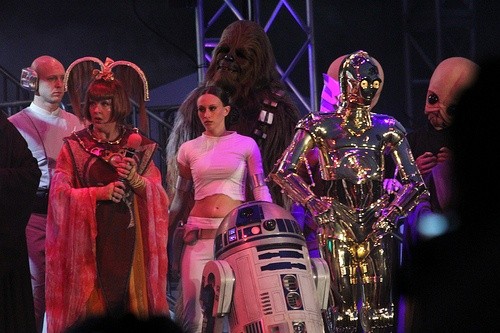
[20,67,37,92]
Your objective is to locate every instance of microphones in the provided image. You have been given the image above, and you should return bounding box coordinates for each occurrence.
[115,133,142,199]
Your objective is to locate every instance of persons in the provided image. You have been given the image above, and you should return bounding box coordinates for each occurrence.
[269,50,425,333]
[401,55,482,299]
[46,57,170,333]
[9,57,89,333]
[0,112,41,333]
[169,21,297,209]
[168,86,272,333]
